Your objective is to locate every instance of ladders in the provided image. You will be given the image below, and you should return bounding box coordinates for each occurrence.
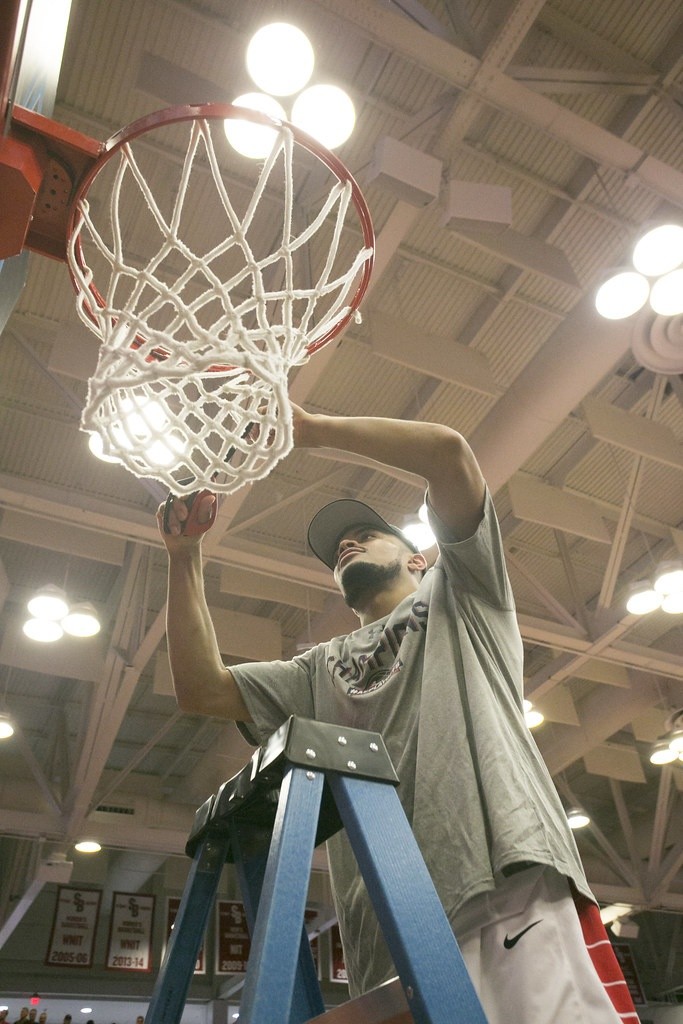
[130,711,498,1023]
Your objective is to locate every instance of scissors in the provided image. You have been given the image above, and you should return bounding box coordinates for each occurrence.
[162,419,260,537]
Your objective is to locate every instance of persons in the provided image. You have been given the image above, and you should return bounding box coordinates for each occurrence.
[0,1007,144,1024]
[155,399,623,1024]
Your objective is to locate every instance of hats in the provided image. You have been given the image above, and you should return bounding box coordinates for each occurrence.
[306,497,429,575]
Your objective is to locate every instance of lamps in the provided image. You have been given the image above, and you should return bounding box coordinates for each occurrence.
[574,161,683,321]
[608,443,683,615]
[21,555,102,643]
[610,917,639,938]
[560,771,590,828]
[0,665,15,738]
[648,673,683,766]
